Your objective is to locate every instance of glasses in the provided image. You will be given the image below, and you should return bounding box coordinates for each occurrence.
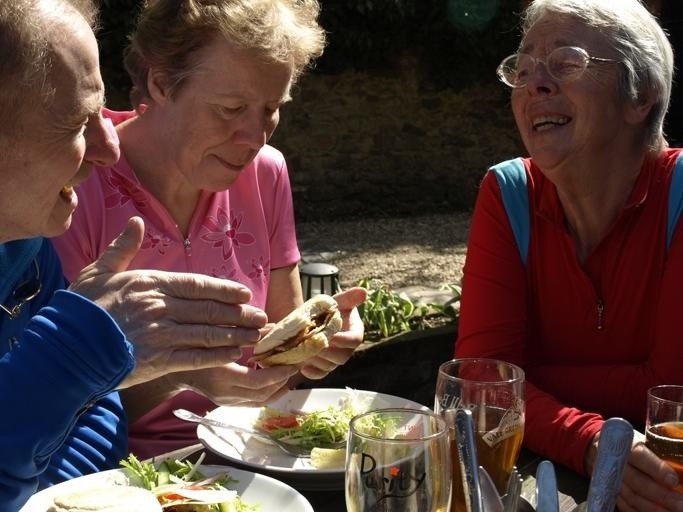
[495,46,618,89]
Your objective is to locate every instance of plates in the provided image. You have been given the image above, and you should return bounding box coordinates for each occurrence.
[196,385,441,477]
[15,459,317,512]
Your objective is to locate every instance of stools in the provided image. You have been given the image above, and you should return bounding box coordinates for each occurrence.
[300,263,340,301]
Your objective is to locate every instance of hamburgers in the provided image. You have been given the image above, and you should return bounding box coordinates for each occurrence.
[252,294,344,369]
[53,486,163,512]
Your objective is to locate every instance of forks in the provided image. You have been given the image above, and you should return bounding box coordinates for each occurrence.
[501,463,524,510]
[171,405,354,459]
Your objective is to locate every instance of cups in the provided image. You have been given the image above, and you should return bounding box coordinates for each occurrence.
[644,383,683,495]
[433,355,528,512]
[343,407,455,512]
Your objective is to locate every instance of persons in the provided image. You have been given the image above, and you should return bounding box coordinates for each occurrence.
[49,2,461,512]
[451,0,681,509]
[0,3,268,512]
